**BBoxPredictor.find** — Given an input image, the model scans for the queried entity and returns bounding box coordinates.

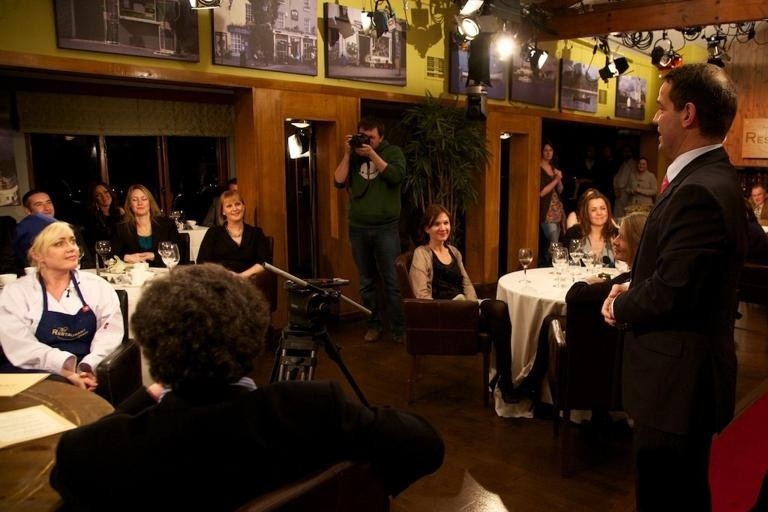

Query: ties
[660,174,670,194]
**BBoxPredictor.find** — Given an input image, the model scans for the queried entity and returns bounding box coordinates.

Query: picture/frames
[53,0,201,67]
[559,56,602,114]
[510,41,557,107]
[447,26,507,99]
[614,71,648,122]
[325,3,406,86]
[212,0,319,78]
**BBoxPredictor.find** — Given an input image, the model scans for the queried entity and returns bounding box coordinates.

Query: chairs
[548,314,627,479]
[97,289,142,404]
[699,377,768,512]
[245,463,364,512]
[393,250,495,409]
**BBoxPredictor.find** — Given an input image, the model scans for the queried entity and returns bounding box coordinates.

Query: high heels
[504,382,541,412]
[490,373,519,404]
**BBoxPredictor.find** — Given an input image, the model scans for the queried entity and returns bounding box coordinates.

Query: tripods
[270,332,377,420]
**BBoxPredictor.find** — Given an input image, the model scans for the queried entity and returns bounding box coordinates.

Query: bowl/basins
[186,221,196,226]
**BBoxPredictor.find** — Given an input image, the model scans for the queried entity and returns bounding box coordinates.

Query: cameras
[350,135,370,148]
[284,278,351,329]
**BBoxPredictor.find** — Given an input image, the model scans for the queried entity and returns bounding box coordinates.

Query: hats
[13,213,58,255]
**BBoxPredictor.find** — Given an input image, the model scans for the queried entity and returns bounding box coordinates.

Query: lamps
[599,42,630,84]
[287,119,311,159]
[529,49,549,72]
[451,18,480,51]
[652,27,685,79]
[459,0,484,17]
[361,0,393,40]
[701,25,731,66]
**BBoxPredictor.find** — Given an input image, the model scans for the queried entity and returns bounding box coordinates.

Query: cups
[157,242,170,269]
[134,262,148,270]
[552,246,568,288]
[161,243,180,271]
[24,267,36,275]
[0,274,16,289]
[126,270,146,288]
[569,239,584,275]
[581,245,594,276]
[549,241,561,256]
[95,240,111,269]
[519,248,533,284]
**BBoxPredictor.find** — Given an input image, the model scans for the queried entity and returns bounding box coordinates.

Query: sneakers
[364,329,388,342]
[394,335,403,347]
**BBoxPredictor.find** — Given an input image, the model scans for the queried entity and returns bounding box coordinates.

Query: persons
[537,141,564,267]
[409,203,523,404]
[565,194,620,268]
[197,190,272,291]
[21,190,54,217]
[110,184,187,267]
[82,181,126,268]
[48,262,446,512]
[750,184,768,229]
[601,63,749,512]
[613,147,637,218]
[510,212,650,413]
[625,156,659,207]
[202,178,237,228]
[565,188,603,230]
[0,212,125,392]
[332,114,407,344]
